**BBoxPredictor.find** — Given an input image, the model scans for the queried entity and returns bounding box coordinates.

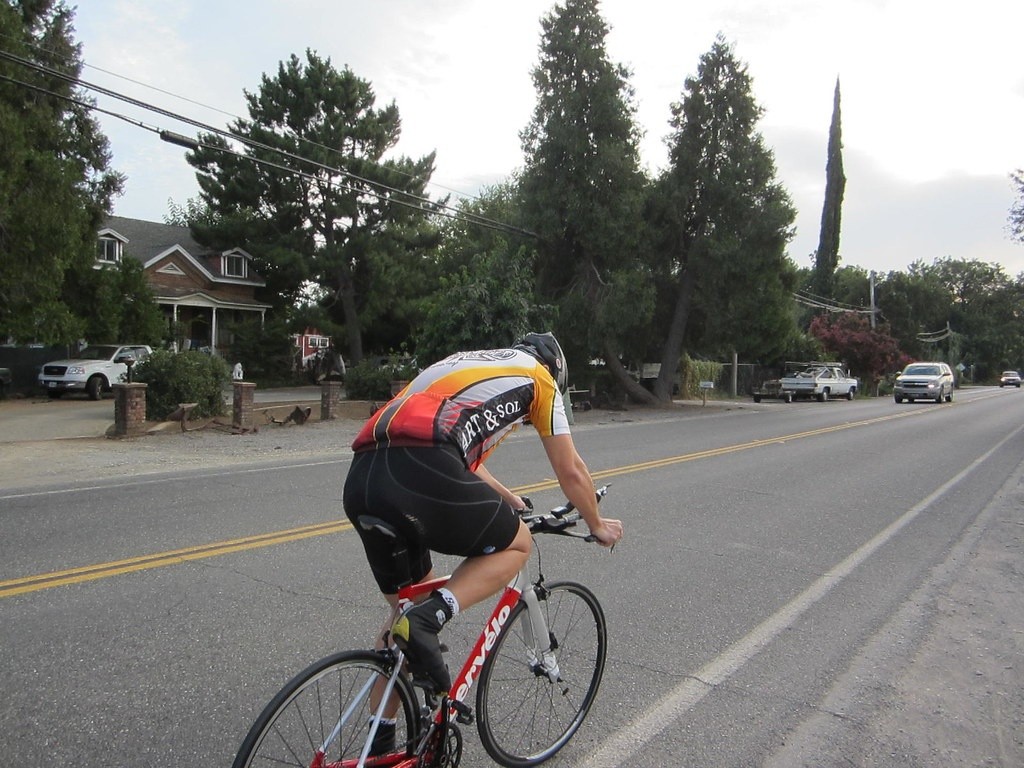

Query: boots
[389,591,454,694]
[360,724,398,768]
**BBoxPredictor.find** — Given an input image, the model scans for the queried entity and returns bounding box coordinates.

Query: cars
[997,369,1021,386]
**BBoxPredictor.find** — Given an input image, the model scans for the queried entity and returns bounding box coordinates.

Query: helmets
[511,331,569,396]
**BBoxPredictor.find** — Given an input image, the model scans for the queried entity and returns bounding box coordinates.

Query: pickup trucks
[37,343,155,402]
[752,367,857,403]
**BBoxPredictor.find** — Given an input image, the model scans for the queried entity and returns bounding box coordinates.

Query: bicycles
[227,482,624,768]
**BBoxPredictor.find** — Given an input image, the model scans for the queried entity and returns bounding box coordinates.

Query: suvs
[892,361,955,403]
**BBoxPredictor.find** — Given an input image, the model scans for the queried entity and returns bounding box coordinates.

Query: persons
[342,331,624,768]
